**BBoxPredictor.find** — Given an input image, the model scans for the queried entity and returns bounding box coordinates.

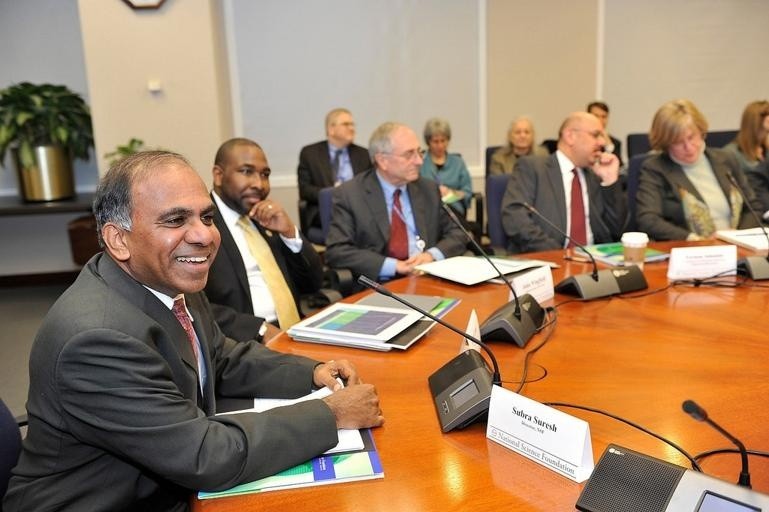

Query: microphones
[575,400,769,512]
[440,202,546,348]
[725,168,768,280]
[518,199,648,301]
[356,275,501,434]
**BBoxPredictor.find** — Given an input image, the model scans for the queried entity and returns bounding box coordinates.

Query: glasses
[381,150,426,160]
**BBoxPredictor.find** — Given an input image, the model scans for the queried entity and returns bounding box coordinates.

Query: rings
[267,205,272,208]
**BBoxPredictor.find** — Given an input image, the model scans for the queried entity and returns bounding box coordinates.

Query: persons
[588,104,623,167]
[324,123,467,297]
[1,151,384,511]
[420,119,472,215]
[724,100,769,174]
[488,119,549,179]
[202,139,324,351]
[634,99,763,241]
[501,112,627,253]
[295,108,372,246]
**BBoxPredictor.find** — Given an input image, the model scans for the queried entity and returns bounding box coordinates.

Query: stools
[68,218,105,266]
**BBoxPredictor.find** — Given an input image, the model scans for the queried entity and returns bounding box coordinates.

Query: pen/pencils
[563,256,593,262]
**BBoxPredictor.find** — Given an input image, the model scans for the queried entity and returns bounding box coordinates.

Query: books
[286,292,462,351]
[197,385,384,501]
[716,226,768,252]
[570,240,669,270]
[415,251,562,287]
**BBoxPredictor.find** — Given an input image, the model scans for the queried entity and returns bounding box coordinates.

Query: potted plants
[0,81,94,202]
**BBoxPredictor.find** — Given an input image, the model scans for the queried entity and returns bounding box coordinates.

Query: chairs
[628,152,655,211]
[0,395,31,512]
[298,199,308,235]
[324,268,354,296]
[486,146,500,176]
[486,174,511,249]
[464,191,483,245]
[626,133,651,156]
[544,139,558,155]
[706,130,738,151]
[298,287,343,318]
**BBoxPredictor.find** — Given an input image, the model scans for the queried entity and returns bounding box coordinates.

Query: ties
[331,149,343,175]
[170,297,199,362]
[566,167,587,249]
[389,188,408,261]
[235,214,301,331]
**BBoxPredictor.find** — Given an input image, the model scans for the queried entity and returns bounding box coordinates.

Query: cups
[621,231,649,272]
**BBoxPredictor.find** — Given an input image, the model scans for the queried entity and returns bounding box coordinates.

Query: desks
[190,239,769,512]
